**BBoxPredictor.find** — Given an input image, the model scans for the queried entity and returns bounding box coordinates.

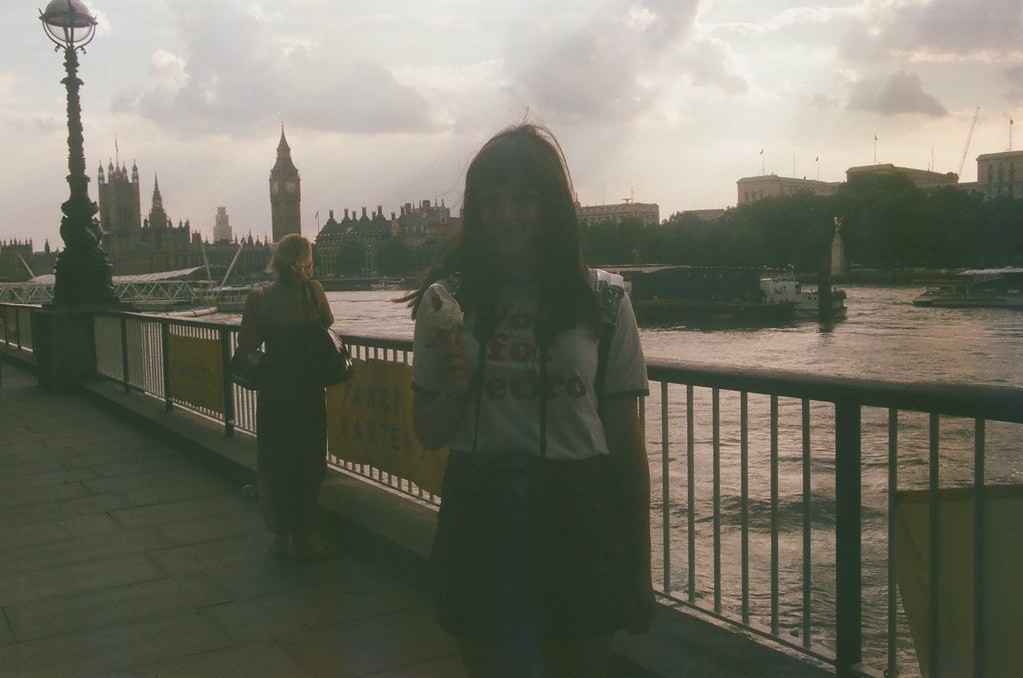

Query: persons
[393,125,650,678]
[238,234,335,561]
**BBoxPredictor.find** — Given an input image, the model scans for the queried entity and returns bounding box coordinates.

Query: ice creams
[423,283,463,344]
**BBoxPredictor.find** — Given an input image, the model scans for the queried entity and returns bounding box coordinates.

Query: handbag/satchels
[227,345,277,392]
[305,279,353,387]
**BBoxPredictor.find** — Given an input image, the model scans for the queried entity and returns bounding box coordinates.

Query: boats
[913,265,1023,309]
[216,279,252,312]
[603,262,794,324]
[759,265,848,315]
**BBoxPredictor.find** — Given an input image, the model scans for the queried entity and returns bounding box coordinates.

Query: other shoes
[293,536,324,554]
[268,541,288,560]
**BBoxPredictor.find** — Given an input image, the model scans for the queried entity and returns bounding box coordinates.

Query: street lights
[37,0,128,311]
